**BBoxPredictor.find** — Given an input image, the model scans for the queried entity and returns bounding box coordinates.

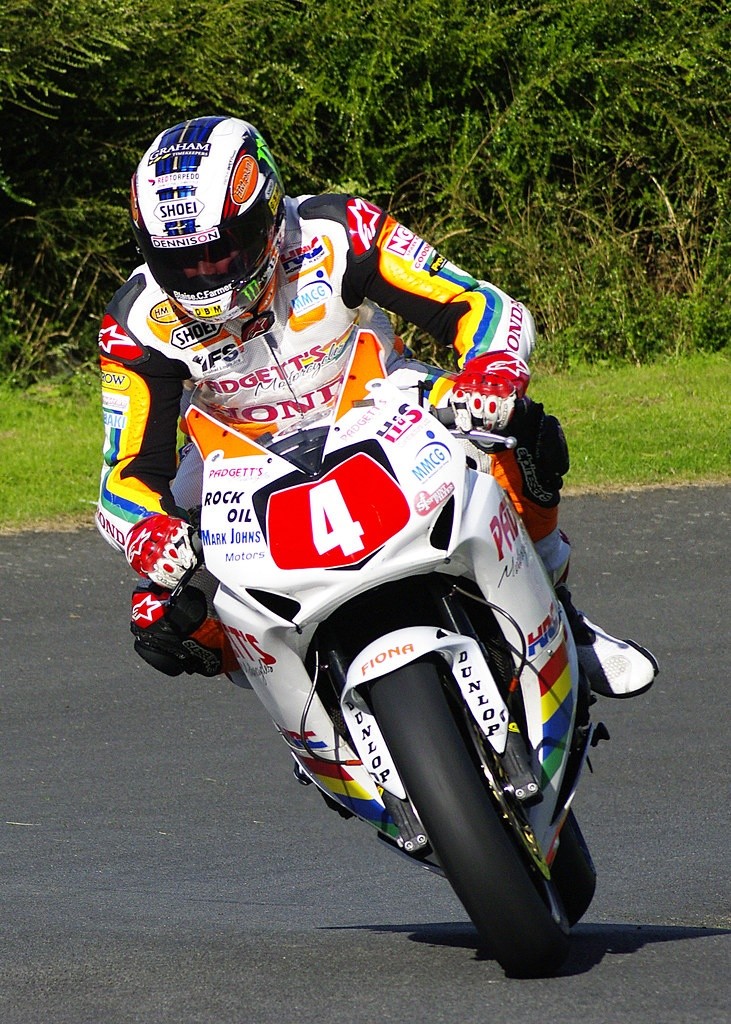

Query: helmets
[130,115,286,325]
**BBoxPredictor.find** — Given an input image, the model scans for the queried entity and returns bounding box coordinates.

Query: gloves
[123,515,203,590]
[447,349,531,432]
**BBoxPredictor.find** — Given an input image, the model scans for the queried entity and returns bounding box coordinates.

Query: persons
[93,111,663,706]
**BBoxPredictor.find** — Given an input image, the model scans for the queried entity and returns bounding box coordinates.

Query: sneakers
[567,612,659,700]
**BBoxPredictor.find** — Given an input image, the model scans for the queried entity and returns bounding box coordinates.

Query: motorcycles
[131,318,613,979]
